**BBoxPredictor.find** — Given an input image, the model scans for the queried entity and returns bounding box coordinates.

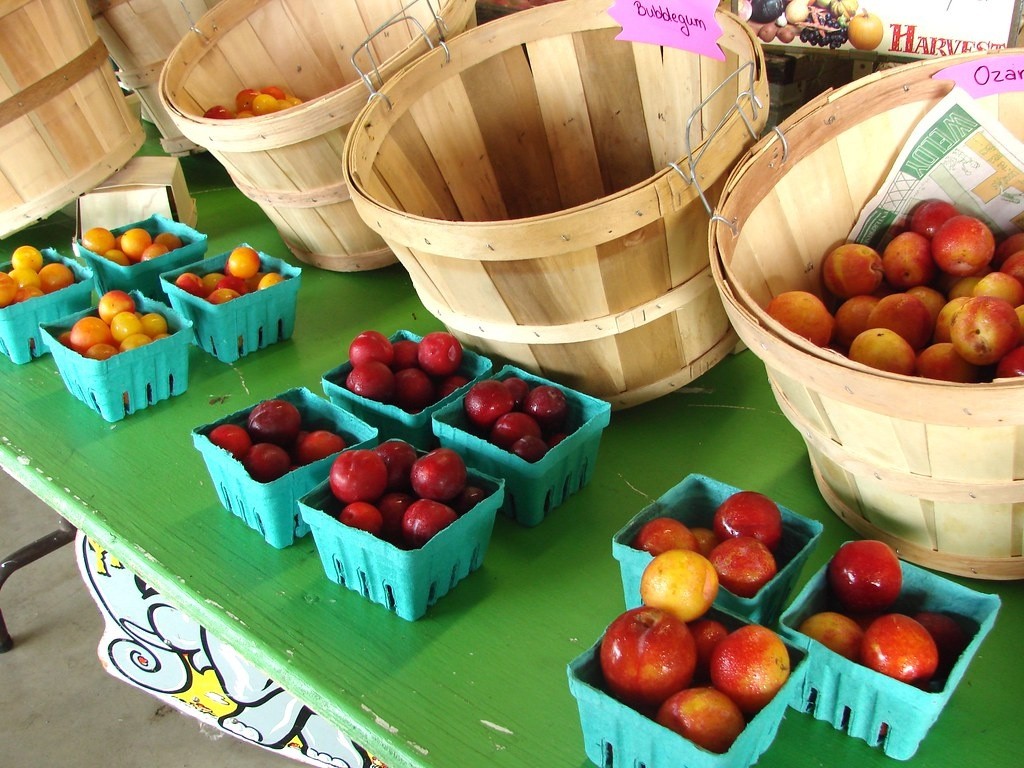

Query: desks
[0,102,1024,768]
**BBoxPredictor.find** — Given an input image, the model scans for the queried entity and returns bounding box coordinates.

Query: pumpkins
[848,7,884,51]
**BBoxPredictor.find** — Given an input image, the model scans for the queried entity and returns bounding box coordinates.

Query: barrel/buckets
[84,0,225,159]
[349,0,770,412]
[0,0,149,242]
[690,49,1024,580]
[157,0,478,273]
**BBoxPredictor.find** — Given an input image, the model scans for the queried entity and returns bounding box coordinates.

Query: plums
[768,202,1024,384]
[204,89,304,117]
[0,228,285,359]
[600,491,964,756]
[211,331,569,546]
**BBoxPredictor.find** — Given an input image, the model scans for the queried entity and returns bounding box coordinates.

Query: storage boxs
[75,158,198,243]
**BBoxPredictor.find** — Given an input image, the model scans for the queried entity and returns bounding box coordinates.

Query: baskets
[611,472,824,627]
[296,438,505,622]
[158,242,302,365]
[566,603,810,768]
[37,288,188,423]
[774,540,1002,761]
[192,385,378,549]
[0,245,93,364]
[321,330,492,454]
[431,364,612,526]
[73,213,206,308]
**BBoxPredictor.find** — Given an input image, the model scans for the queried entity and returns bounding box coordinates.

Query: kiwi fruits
[747,24,795,44]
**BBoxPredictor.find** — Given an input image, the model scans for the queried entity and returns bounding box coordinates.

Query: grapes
[799,13,848,50]
